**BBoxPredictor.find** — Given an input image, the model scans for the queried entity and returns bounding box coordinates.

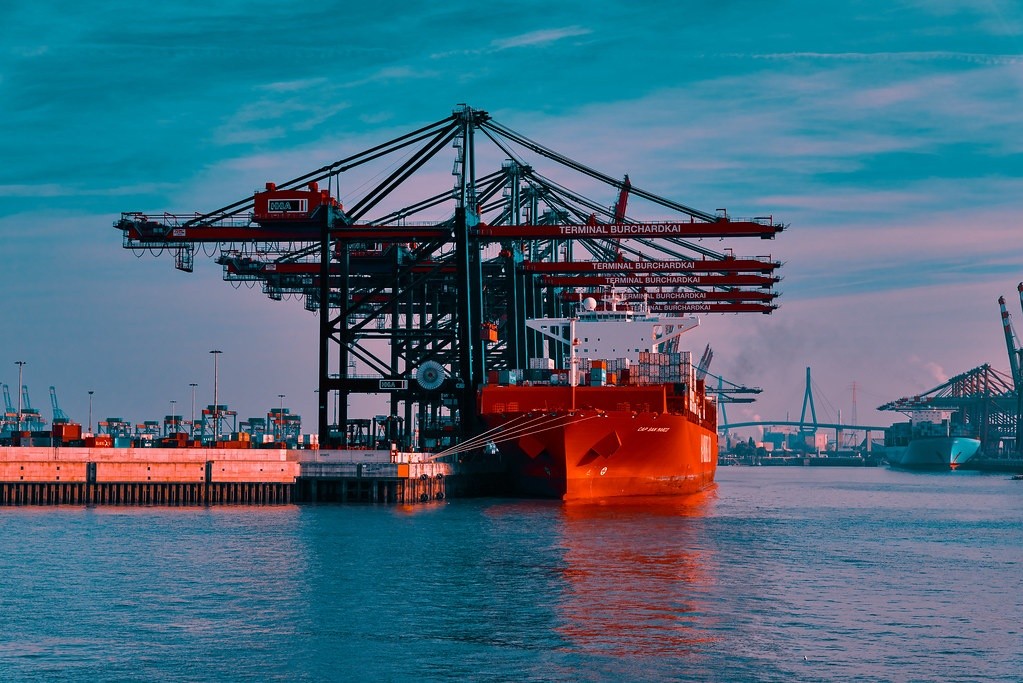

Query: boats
[880,435,981,473]
[476,315,723,506]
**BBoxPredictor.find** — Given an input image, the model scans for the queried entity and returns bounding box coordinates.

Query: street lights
[88,390,94,433]
[170,400,176,433]
[330,373,339,432]
[14,361,26,418]
[278,395,285,436]
[189,383,199,437]
[208,349,223,415]
